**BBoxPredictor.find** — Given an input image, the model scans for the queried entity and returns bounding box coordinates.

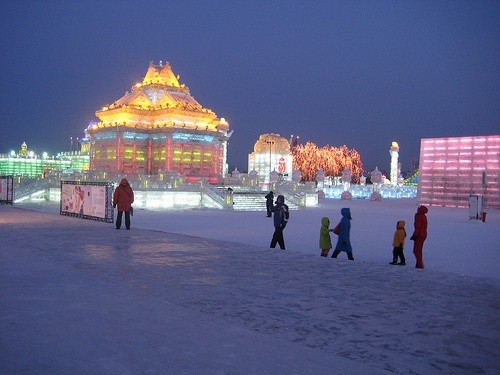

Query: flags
[294,140,297,147]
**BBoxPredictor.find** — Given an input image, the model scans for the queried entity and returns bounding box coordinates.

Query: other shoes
[397,260,406,265]
[116,227,120,229]
[126,228,130,230]
[389,259,398,265]
[266,216,271,217]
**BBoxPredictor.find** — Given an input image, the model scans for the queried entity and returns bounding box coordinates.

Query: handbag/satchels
[130,207,133,217]
[333,223,340,235]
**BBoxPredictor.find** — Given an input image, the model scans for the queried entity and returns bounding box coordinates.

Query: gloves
[344,241,348,247]
[398,243,403,248]
[410,234,417,241]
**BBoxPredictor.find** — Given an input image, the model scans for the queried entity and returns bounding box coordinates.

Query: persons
[319,217,334,257]
[332,207,353,261]
[389,220,407,266]
[113,177,135,230]
[410,205,428,269]
[228,187,235,206]
[265,191,274,218]
[270,195,289,250]
